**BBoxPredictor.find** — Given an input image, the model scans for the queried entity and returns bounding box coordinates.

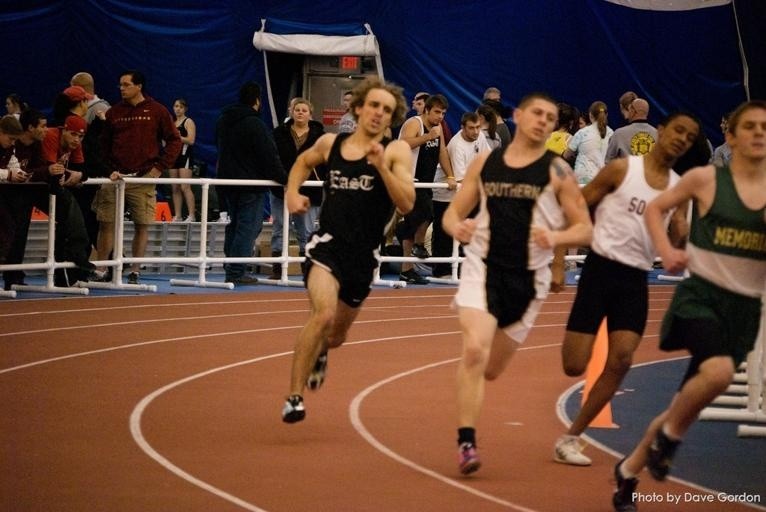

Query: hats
[62,85,95,102]
[57,115,88,134]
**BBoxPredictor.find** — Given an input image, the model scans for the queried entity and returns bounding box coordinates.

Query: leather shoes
[224,275,259,286]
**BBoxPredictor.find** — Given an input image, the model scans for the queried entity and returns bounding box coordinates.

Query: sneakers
[612,456,642,512]
[399,268,430,285]
[128,271,140,284]
[551,434,593,466]
[281,393,307,424]
[643,420,684,484]
[455,443,482,475]
[305,350,328,391]
[409,243,431,259]
[184,214,195,224]
[172,216,182,222]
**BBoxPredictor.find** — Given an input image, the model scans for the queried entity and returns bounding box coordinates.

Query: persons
[216,82,289,285]
[413,94,454,257]
[480,99,511,144]
[483,87,501,102]
[561,100,614,185]
[612,102,766,511]
[416,94,453,146]
[35,116,97,287]
[165,98,197,222]
[619,92,639,124]
[342,91,354,112]
[604,99,659,164]
[706,113,733,165]
[574,113,590,129]
[550,110,706,465]
[719,113,736,170]
[398,95,457,284]
[1,110,71,291]
[339,98,367,133]
[544,102,580,158]
[406,92,430,119]
[70,73,130,282]
[474,88,502,117]
[282,97,320,240]
[475,105,501,150]
[0,116,34,294]
[579,110,591,129]
[91,70,183,285]
[432,112,492,275]
[268,99,327,284]
[443,94,593,473]
[5,93,32,131]
[281,76,416,423]
[62,86,110,280]
